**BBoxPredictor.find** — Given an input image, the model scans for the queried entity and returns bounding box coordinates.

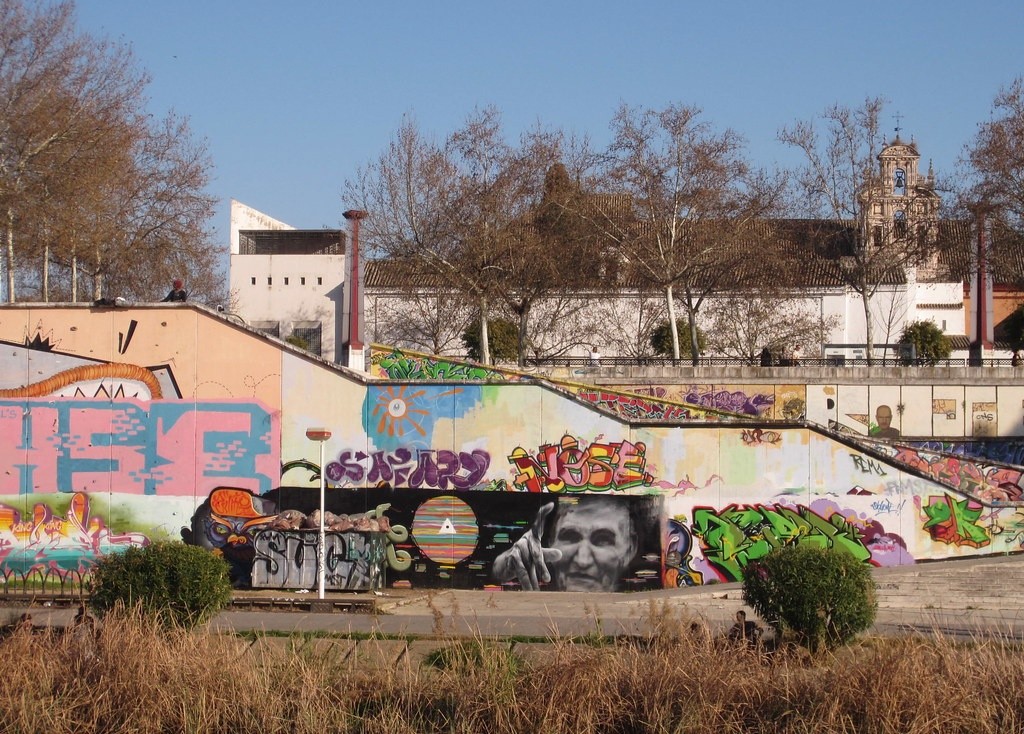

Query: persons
[159,279,188,303]
[724,610,765,650]
